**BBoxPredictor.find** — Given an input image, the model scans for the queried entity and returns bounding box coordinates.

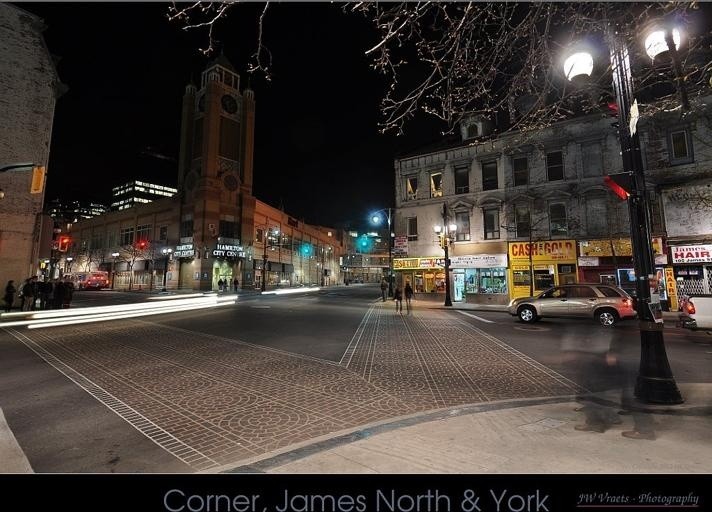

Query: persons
[380,280,389,303]
[217,279,224,290]
[606,299,661,441]
[573,342,633,431]
[234,278,239,292]
[393,283,404,316]
[230,279,233,291]
[4,273,76,315]
[223,279,228,291]
[403,283,414,316]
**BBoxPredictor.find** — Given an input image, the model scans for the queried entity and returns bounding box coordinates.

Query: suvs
[79,273,108,291]
[506,283,637,327]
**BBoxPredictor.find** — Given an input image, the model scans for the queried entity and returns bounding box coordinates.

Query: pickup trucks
[676,293,712,336]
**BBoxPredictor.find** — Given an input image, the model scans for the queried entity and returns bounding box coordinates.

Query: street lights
[162,248,172,292]
[109,252,120,291]
[563,23,684,402]
[361,207,393,297]
[433,200,459,306]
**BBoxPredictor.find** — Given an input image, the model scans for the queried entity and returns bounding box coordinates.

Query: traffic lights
[59,237,67,252]
[438,235,441,246]
[603,170,638,203]
[304,243,309,254]
[140,240,145,249]
